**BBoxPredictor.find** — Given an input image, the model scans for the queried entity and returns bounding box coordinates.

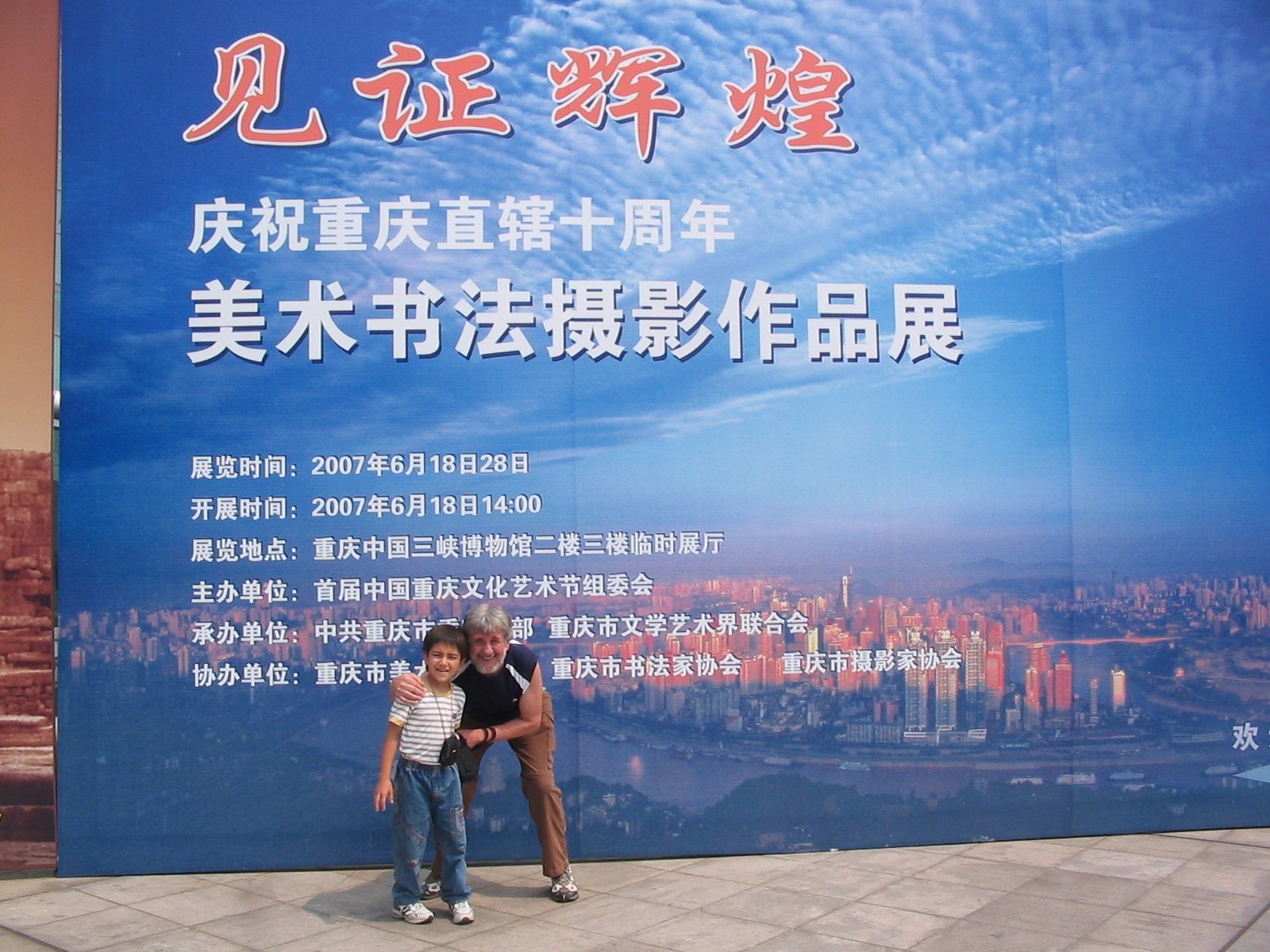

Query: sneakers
[551,864,578,902]
[392,898,434,925]
[449,900,474,925]
[417,873,441,900]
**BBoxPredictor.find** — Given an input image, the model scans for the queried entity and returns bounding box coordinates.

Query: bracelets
[488,727,496,743]
[483,728,488,744]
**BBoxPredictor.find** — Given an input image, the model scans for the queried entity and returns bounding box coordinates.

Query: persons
[375,625,473,926]
[391,605,578,902]
